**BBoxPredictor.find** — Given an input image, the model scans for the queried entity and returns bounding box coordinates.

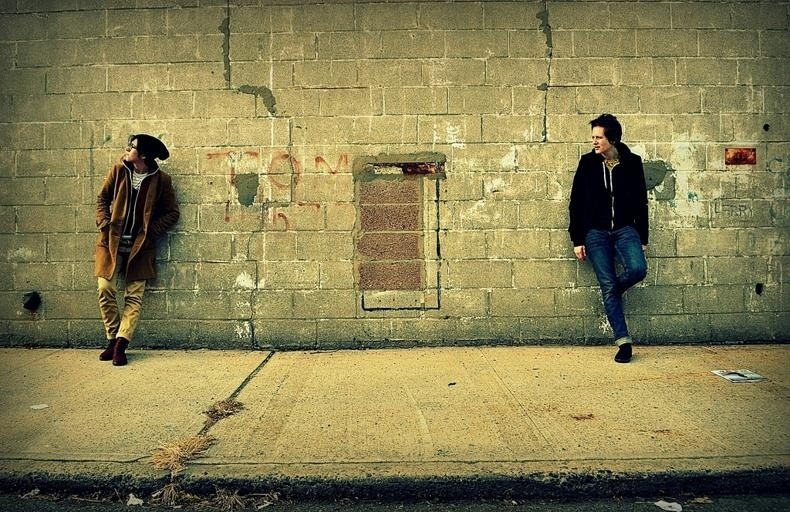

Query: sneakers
[615,343,632,363]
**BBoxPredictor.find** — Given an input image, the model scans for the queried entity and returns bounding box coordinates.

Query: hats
[131,134,169,160]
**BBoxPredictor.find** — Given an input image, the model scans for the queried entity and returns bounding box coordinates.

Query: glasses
[129,143,137,149]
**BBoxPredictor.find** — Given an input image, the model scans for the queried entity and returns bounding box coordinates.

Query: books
[712,369,764,382]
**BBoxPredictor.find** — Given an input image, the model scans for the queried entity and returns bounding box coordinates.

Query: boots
[99,338,117,361]
[112,337,129,366]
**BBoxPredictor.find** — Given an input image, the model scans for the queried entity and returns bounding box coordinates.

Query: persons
[94,134,179,366]
[567,113,649,362]
